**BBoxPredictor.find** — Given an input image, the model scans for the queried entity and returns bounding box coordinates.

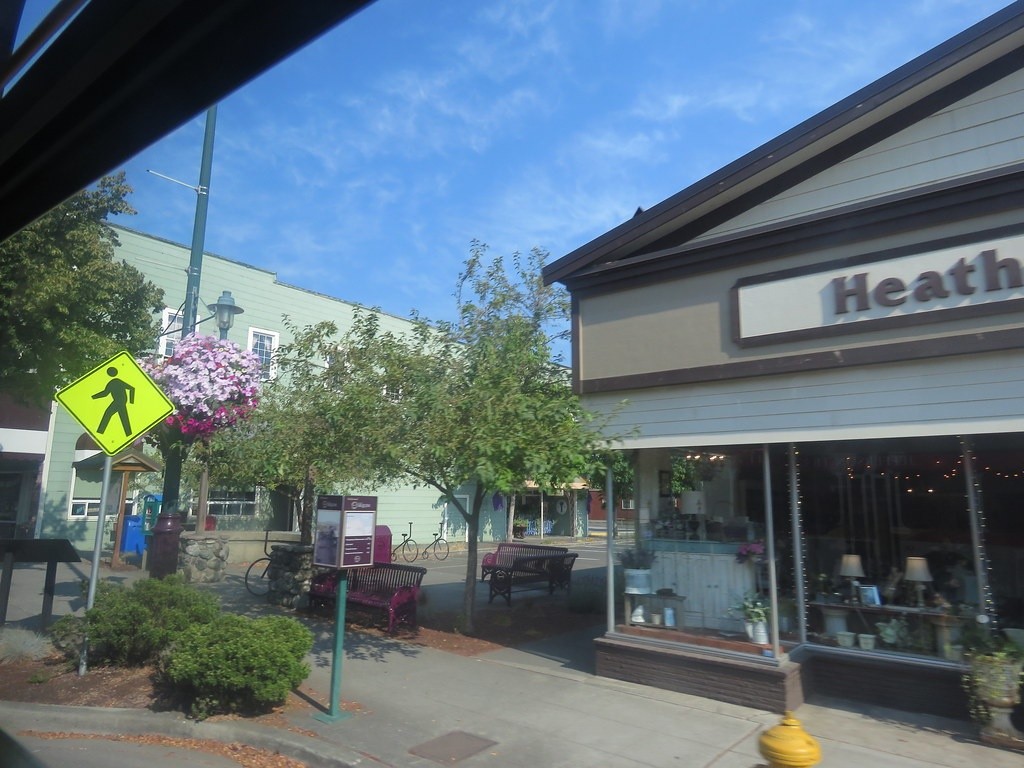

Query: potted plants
[958,639,1024,749]
[615,540,659,595]
[721,590,795,644]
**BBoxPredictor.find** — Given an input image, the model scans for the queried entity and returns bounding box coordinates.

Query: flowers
[735,543,767,564]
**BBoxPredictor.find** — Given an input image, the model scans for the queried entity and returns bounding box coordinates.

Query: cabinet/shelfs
[621,593,687,632]
[644,552,757,631]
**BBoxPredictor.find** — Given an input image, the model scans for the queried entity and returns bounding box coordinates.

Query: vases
[651,611,663,625]
[835,630,857,647]
[856,633,877,650]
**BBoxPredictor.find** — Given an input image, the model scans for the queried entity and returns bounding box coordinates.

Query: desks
[810,603,975,659]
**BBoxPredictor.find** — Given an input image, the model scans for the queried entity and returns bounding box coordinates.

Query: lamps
[902,555,936,607]
[839,553,867,605]
[681,489,708,540]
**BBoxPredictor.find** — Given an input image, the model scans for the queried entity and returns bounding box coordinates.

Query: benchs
[480,538,579,606]
[304,561,428,635]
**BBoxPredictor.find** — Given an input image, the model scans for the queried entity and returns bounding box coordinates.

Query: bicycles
[421,523,450,561]
[391,522,418,562]
[243,556,273,597]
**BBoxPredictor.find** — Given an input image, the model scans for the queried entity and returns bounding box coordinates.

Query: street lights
[144,289,242,580]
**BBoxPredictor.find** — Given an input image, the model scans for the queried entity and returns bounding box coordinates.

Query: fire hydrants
[753,708,822,768]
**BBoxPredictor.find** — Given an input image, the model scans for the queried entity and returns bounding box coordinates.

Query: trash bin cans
[374,525,393,565]
[120,514,145,554]
[206,516,217,531]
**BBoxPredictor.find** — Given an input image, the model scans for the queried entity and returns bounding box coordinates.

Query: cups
[838,631,857,647]
[858,633,877,650]
[650,613,661,625]
[663,607,676,626]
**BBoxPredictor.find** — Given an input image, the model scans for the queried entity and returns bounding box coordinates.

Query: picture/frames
[658,470,673,498]
